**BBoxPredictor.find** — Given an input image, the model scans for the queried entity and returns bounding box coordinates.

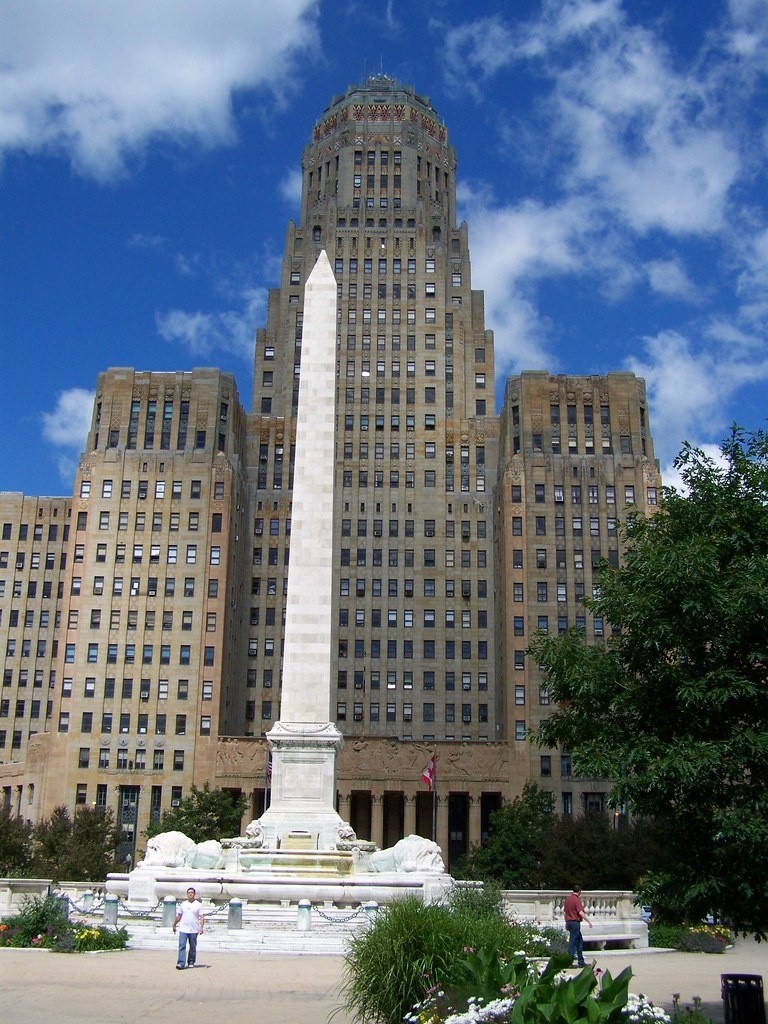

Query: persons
[564,884,592,968]
[173,888,204,970]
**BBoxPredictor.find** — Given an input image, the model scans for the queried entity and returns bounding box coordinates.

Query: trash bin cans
[719,973,768,1024]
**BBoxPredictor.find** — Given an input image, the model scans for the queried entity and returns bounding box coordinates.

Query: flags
[421,754,435,792]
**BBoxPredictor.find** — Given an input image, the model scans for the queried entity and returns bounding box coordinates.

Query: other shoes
[578,964,588,967]
[565,965,577,969]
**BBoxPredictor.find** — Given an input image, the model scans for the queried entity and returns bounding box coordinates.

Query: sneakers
[189,964,194,968]
[176,965,184,969]
